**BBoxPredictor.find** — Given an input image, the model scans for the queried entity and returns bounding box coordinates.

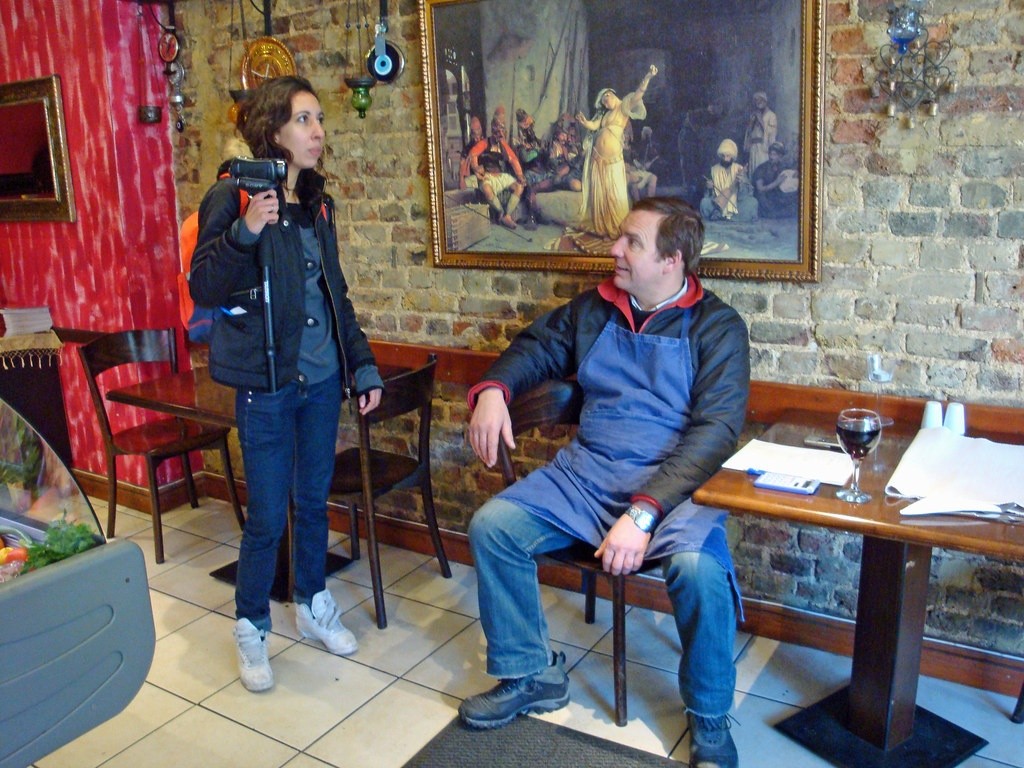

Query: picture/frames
[0,74,76,223]
[418,0,826,283]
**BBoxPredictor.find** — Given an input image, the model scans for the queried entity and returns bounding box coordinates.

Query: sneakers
[459,650,573,728]
[232,617,274,691]
[294,589,358,656]
[682,703,741,768]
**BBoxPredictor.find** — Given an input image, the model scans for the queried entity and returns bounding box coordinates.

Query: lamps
[861,1,958,129]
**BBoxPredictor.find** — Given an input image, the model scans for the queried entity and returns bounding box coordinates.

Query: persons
[188,76,382,691]
[458,198,751,768]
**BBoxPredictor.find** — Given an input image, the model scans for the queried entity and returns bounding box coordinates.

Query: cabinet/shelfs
[0,329,72,466]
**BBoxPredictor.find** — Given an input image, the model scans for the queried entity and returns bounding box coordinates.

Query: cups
[920,401,943,431]
[944,402,966,436]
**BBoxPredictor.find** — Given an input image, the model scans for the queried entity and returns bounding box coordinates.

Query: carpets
[401,714,690,768]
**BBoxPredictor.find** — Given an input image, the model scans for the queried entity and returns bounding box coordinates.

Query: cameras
[231,157,288,200]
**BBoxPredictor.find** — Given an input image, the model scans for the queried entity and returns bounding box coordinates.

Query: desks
[106,363,414,602]
[692,407,1024,768]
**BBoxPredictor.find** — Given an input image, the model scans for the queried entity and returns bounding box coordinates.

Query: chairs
[76,326,246,566]
[497,380,663,728]
[286,352,452,630]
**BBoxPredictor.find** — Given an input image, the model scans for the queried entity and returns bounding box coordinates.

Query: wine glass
[834,408,882,503]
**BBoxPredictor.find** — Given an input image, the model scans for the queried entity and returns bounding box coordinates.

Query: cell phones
[804,435,843,451]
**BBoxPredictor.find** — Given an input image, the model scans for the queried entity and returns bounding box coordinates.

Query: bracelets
[625,507,656,532]
